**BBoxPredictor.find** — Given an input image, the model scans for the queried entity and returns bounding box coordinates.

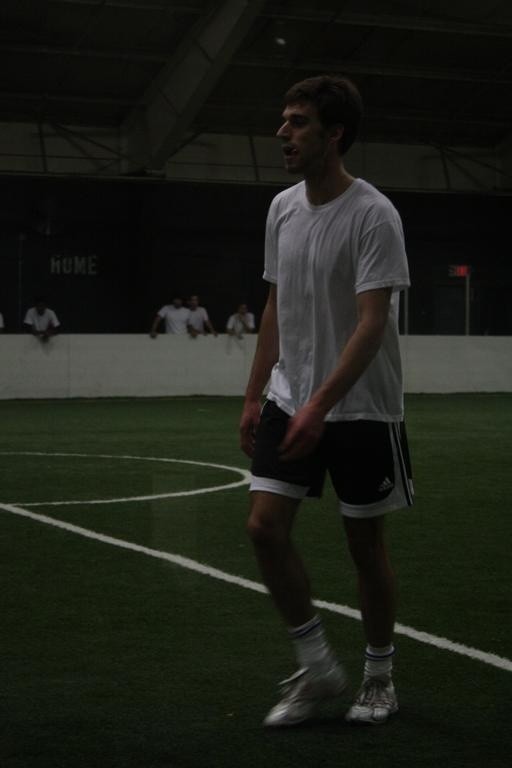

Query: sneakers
[262,659,347,728]
[346,672,401,726]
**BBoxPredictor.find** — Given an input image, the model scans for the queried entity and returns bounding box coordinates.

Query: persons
[148,291,219,339]
[21,298,63,343]
[235,72,413,730]
[226,302,256,338]
[0,311,6,333]
